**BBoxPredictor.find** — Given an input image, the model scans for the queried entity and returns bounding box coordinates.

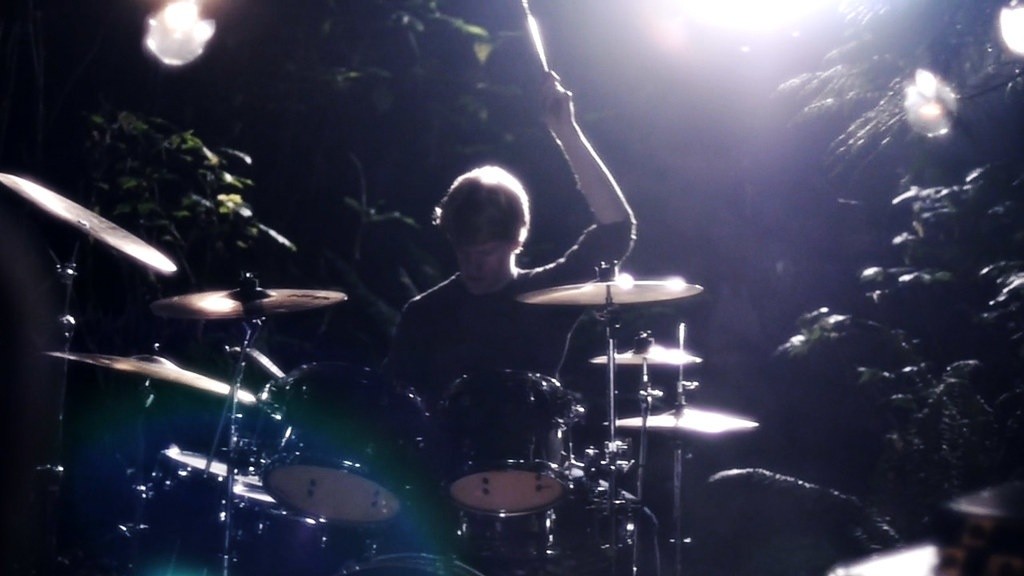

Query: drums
[323,539,488,574]
[248,361,431,524]
[142,449,243,557]
[440,366,579,521]
[220,471,333,575]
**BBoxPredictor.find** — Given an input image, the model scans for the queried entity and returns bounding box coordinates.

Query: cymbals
[0,174,180,277]
[141,285,349,323]
[38,345,257,410]
[515,277,706,306]
[604,408,760,436]
[590,342,703,368]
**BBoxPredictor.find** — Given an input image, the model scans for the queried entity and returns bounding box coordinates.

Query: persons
[379,71,638,576]
